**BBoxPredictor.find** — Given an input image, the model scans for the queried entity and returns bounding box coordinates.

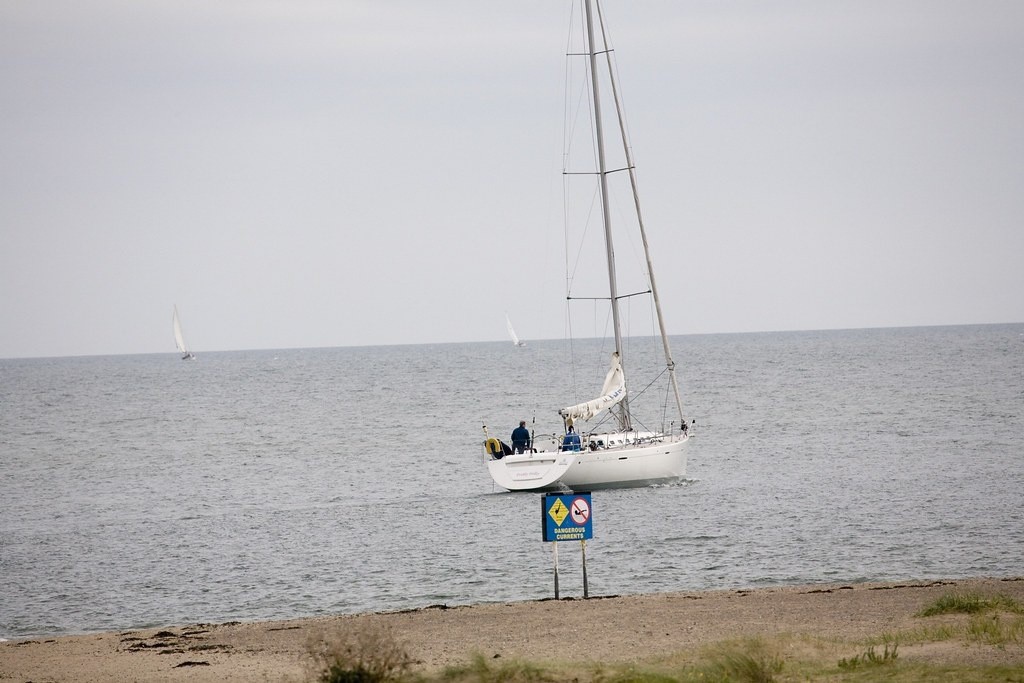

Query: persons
[511,421,530,455]
[563,413,576,434]
[680,419,688,436]
[581,439,605,451]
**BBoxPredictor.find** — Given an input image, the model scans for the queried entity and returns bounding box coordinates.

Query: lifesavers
[486,438,500,454]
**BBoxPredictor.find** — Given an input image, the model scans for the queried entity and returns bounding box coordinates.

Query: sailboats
[478,1,700,495]
[504,314,527,349]
[172,311,196,361]
